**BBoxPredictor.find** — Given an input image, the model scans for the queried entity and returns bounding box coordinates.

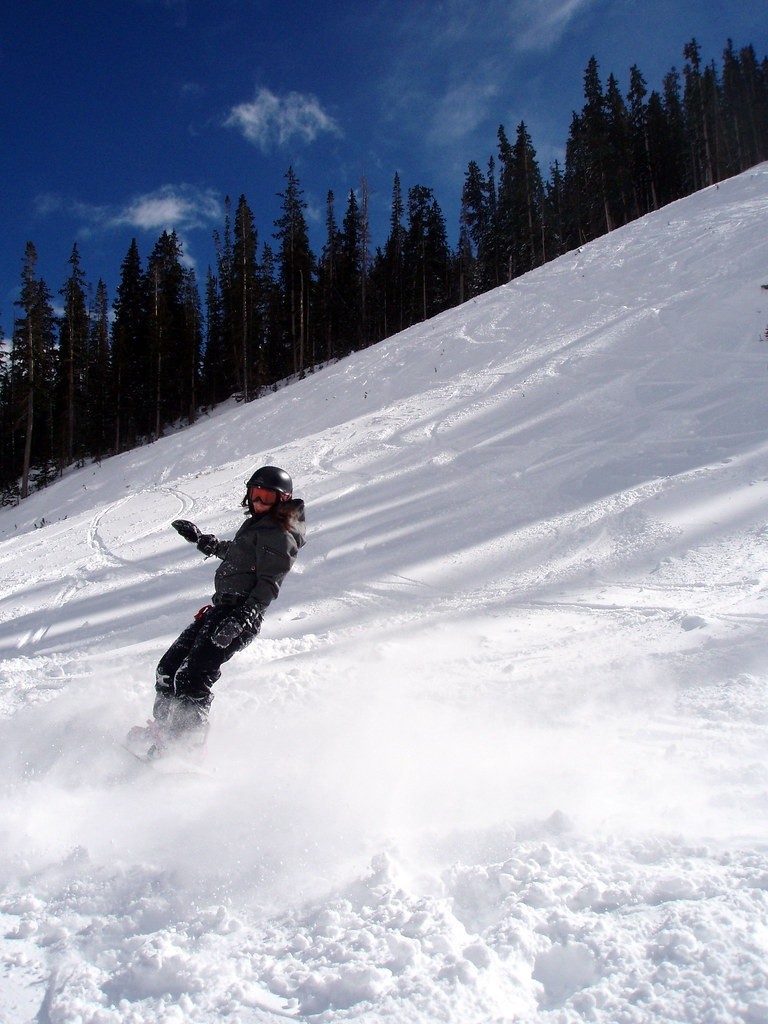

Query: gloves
[212,603,254,649]
[172,519,217,557]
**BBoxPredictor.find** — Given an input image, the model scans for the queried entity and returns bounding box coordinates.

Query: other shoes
[128,719,165,741]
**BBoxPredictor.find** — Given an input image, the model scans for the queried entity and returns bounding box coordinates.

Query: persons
[147,467,305,753]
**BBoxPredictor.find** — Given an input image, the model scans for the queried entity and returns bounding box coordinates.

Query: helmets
[247,465,293,497]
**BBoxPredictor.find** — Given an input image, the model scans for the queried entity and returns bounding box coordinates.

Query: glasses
[247,486,281,505]
